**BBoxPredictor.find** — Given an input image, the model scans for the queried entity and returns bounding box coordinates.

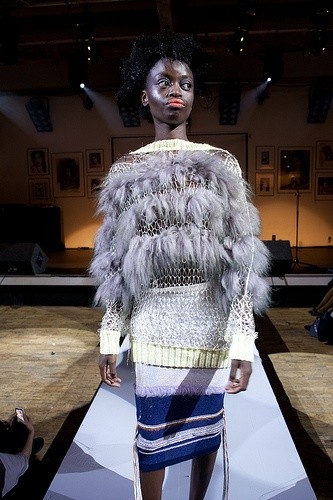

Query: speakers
[261,239,292,277]
[0,243,50,276]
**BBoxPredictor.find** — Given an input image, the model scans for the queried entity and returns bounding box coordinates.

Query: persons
[86,29,270,500]
[304,278,333,345]
[30,151,46,174]
[0,411,35,497]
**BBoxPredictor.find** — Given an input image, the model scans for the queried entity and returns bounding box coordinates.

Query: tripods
[291,181,320,272]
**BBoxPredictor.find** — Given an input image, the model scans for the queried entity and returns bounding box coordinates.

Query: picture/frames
[277,146,313,194]
[87,175,105,198]
[50,151,85,197]
[85,148,105,172]
[315,140,333,170]
[255,145,275,170]
[315,172,333,201]
[255,172,274,196]
[28,177,51,204]
[26,146,49,175]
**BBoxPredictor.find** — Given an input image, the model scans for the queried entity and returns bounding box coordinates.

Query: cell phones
[15,408,24,422]
